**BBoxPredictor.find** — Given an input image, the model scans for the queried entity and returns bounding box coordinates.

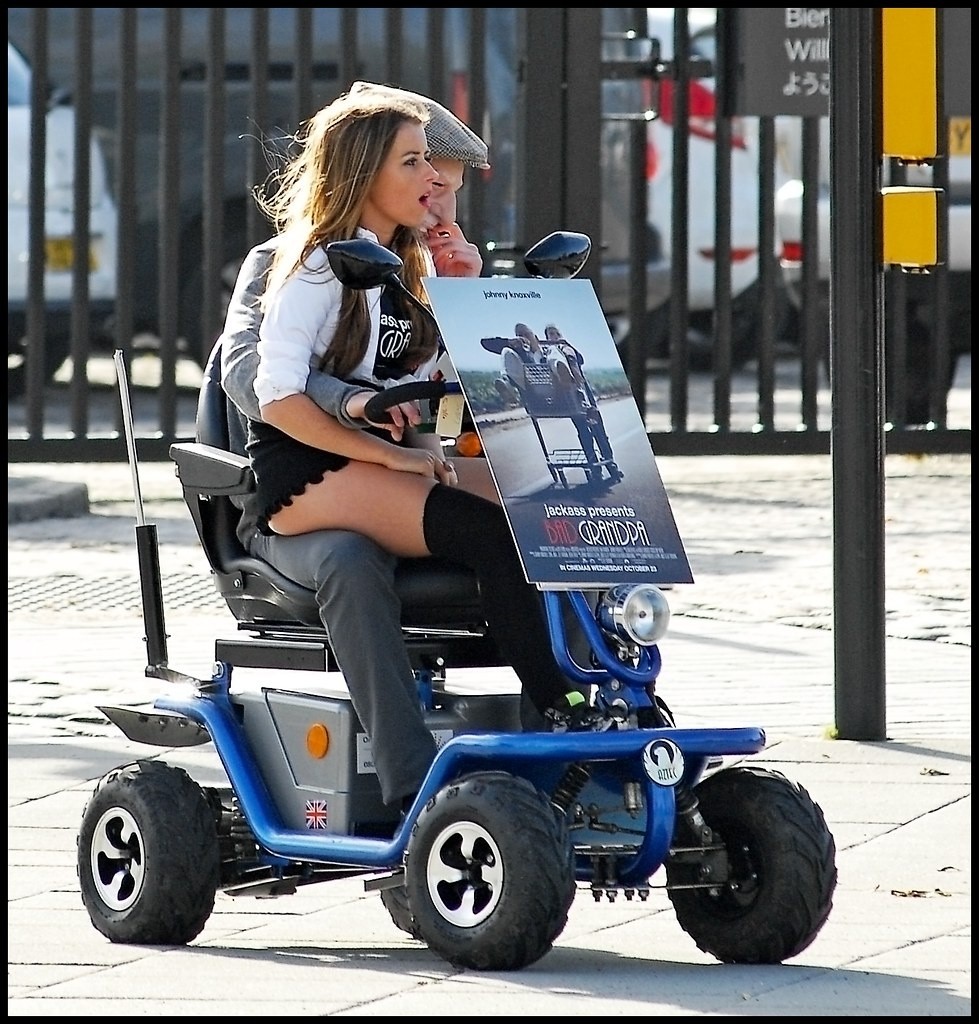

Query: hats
[350,80,489,172]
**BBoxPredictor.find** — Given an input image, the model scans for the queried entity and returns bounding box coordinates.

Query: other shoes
[595,473,602,483]
[613,470,623,480]
[546,691,615,736]
[494,378,521,404]
[575,411,589,425]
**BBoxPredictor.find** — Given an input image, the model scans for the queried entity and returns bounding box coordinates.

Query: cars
[6,44,122,405]
[4,6,973,421]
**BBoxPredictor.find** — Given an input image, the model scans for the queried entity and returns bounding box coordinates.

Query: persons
[243,82,595,732]
[474,322,622,488]
[223,78,608,828]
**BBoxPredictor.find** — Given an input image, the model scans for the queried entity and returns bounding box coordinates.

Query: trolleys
[509,338,621,498]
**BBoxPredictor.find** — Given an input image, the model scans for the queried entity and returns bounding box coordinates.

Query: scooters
[73,233,839,971]
